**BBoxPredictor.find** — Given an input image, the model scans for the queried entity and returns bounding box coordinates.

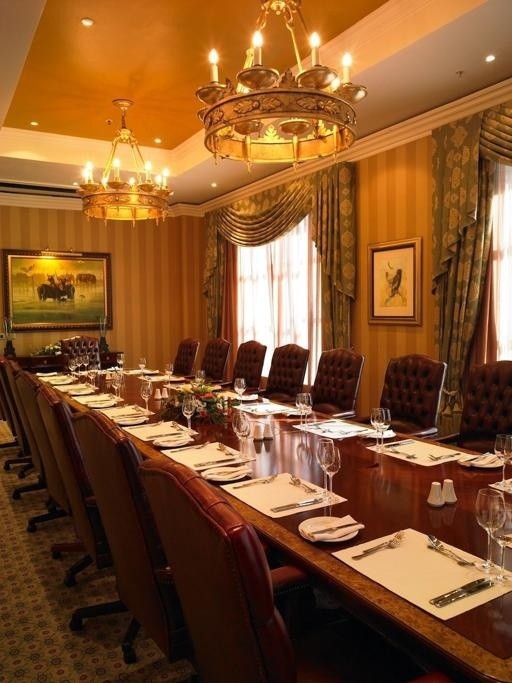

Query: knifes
[310,521,363,532]
[428,577,485,603]
[434,581,494,608]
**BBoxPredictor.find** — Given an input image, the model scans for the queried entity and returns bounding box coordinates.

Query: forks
[351,532,401,561]
[37,372,73,385]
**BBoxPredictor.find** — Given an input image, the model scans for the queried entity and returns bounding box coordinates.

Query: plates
[115,416,146,425]
[89,401,118,409]
[202,466,246,481]
[298,516,358,542]
[153,437,188,448]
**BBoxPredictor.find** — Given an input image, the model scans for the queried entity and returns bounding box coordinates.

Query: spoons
[426,534,474,566]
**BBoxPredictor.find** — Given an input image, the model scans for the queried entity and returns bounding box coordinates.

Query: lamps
[196,0,367,174]
[76,99,169,228]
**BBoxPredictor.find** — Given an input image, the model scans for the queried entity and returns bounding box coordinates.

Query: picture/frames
[368,235,422,326]
[0,248,113,333]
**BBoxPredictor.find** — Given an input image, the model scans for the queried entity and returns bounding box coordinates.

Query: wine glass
[234,378,247,404]
[183,394,197,428]
[319,447,341,492]
[491,504,511,585]
[296,393,305,425]
[315,438,335,489]
[375,409,391,444]
[234,417,250,454]
[504,435,512,492]
[140,381,153,410]
[231,412,246,452]
[182,400,195,431]
[494,434,508,487]
[476,489,507,572]
[370,408,385,445]
[301,392,313,423]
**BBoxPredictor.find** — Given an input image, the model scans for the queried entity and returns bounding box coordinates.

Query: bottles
[427,482,445,507]
[441,479,458,505]
